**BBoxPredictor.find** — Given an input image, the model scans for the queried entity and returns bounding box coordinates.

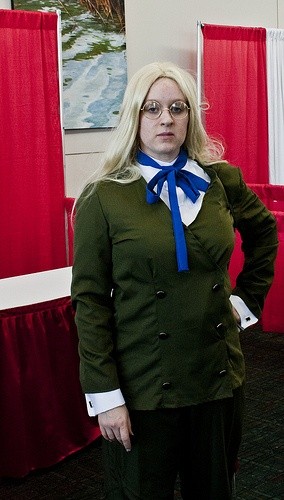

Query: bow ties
[142,165,208,272]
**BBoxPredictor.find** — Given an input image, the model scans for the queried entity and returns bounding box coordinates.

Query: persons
[71,63,278,500]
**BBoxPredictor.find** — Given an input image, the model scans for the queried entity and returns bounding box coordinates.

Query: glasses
[140,100,191,119]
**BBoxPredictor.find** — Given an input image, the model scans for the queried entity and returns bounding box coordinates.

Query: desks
[0,265,102,478]
